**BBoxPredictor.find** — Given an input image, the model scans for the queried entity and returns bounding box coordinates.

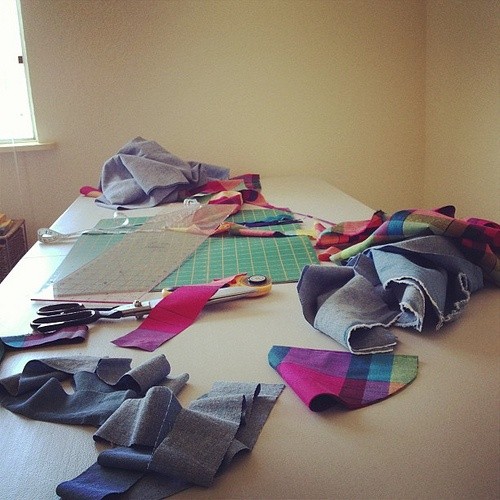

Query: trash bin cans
[0,212,28,282]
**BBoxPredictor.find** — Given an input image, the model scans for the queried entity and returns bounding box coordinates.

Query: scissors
[31,285,258,331]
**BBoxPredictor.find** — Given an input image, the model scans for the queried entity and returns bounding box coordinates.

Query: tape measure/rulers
[36,197,204,243]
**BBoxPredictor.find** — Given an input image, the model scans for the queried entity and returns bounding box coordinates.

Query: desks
[1,169,500,500]
